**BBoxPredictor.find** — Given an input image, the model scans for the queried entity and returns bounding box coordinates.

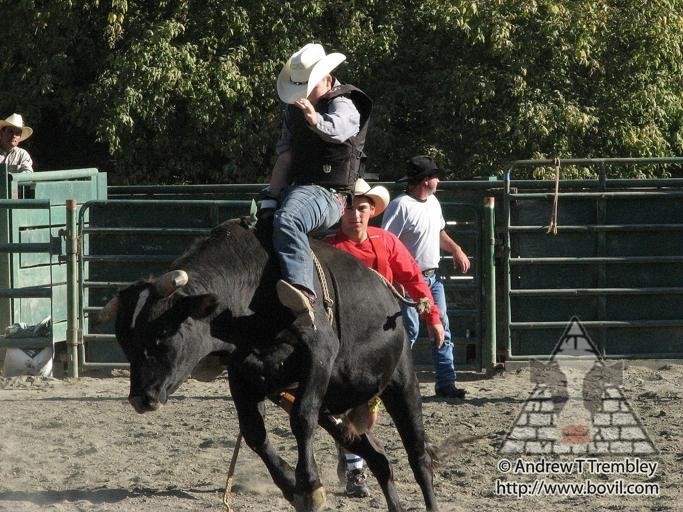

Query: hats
[0,113,33,142]
[355,178,390,218]
[393,155,452,181]
[276,43,347,104]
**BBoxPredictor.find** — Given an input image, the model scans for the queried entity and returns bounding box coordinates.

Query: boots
[436,383,464,398]
[275,279,316,327]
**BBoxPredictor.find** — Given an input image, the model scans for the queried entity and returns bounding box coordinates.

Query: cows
[99,217,438,512]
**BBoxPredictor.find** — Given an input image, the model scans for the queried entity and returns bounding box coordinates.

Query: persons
[380,155,471,397]
[320,178,445,498]
[256,43,373,328]
[0,113,34,173]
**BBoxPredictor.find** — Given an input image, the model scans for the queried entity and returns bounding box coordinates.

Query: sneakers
[337,453,369,497]
